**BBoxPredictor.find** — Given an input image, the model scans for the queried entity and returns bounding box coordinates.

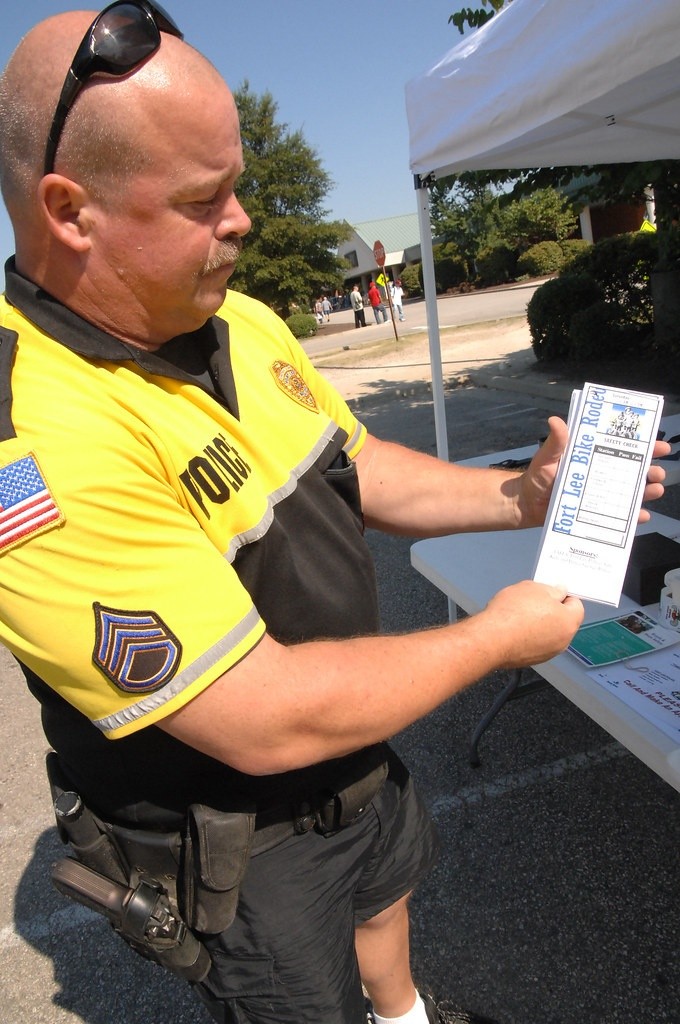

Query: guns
[48,854,213,984]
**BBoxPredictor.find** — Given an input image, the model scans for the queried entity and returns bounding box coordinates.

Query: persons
[314,279,407,328]
[0,1,673,1024]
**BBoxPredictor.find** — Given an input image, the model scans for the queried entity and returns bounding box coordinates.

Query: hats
[395,280,401,286]
[370,282,375,287]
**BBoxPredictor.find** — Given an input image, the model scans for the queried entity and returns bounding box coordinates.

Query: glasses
[43,1,184,175]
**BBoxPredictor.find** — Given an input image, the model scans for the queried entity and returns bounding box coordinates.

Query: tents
[405,0,679,624]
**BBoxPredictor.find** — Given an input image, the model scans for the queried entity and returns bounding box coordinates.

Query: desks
[410,413,680,793]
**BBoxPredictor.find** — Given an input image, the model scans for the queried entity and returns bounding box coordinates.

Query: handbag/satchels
[317,313,322,319]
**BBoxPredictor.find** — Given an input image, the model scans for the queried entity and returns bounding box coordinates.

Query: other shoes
[401,318,406,322]
[367,991,476,1024]
[385,319,391,324]
